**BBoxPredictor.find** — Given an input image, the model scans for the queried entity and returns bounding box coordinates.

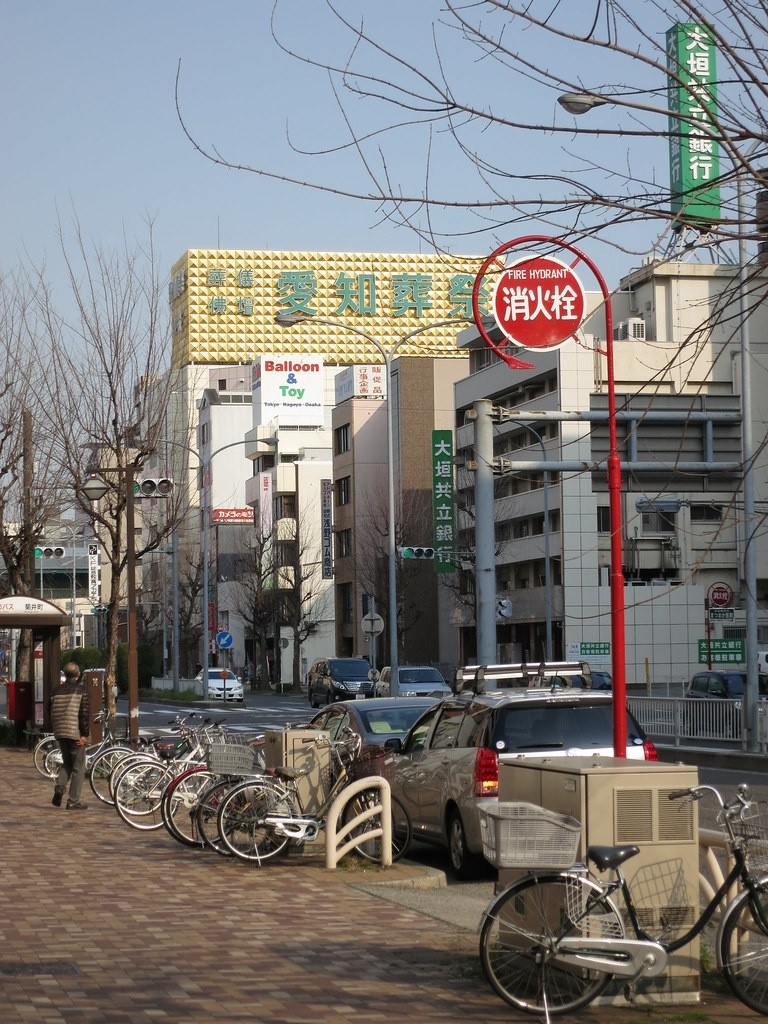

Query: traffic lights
[399,547,435,559]
[131,478,175,497]
[35,546,66,559]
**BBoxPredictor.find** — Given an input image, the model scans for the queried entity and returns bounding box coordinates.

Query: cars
[194,667,245,704]
[377,659,660,872]
[679,671,768,741]
[376,665,453,701]
[301,695,446,791]
[305,656,378,707]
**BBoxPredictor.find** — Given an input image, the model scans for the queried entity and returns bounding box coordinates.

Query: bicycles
[33,708,131,782]
[473,782,767,1024]
[89,711,412,865]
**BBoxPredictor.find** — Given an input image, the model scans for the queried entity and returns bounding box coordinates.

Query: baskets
[476,802,584,869]
[716,801,768,870]
[209,743,255,775]
[331,745,396,786]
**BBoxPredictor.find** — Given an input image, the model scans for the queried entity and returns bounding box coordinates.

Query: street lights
[124,435,280,702]
[20,479,110,598]
[557,92,758,757]
[275,314,496,699]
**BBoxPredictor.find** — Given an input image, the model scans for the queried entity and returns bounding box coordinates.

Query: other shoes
[52,790,63,807]
[67,803,89,809]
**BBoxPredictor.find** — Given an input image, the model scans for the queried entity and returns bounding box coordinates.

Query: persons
[49,661,90,809]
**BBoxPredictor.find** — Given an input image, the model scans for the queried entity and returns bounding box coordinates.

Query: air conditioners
[613,318,646,342]
